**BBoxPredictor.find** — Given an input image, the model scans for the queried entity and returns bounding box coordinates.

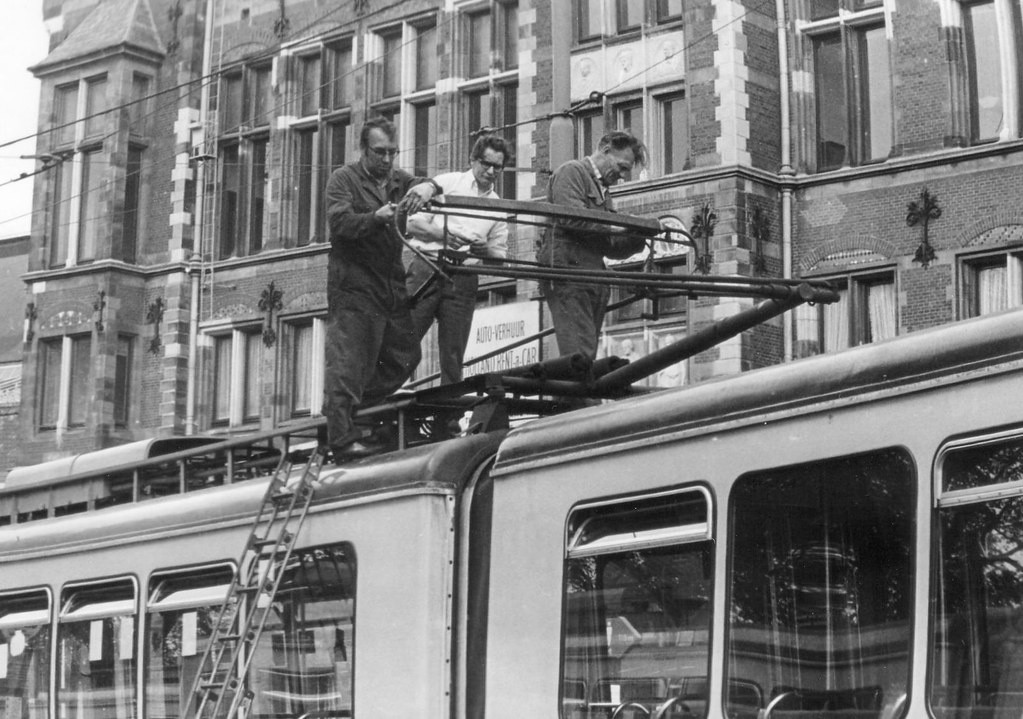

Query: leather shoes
[331,442,383,465]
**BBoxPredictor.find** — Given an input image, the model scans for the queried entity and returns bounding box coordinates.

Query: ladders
[182,442,328,719]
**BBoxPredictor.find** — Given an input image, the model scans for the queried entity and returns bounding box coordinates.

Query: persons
[400,136,509,437]
[537,133,654,410]
[324,115,444,466]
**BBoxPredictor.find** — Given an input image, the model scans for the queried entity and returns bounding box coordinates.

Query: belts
[418,249,459,263]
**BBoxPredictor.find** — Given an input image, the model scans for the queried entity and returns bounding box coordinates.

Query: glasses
[476,158,503,172]
[367,145,399,157]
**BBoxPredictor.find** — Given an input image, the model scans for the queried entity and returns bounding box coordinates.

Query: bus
[0,194,1023,719]
